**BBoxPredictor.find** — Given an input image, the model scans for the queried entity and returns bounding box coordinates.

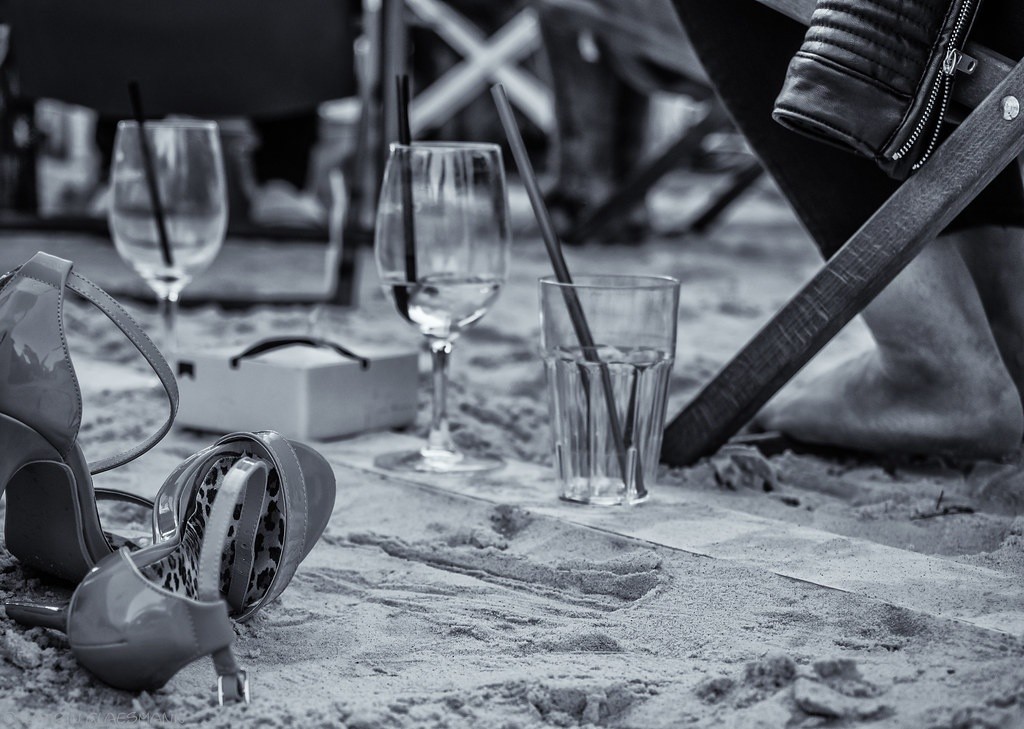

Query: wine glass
[110,122,228,373]
[375,141,512,477]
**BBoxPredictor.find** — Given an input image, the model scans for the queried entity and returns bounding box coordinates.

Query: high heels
[5,430,338,708]
[0,252,181,588]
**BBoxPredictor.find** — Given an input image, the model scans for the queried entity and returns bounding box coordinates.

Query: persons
[674,1,1024,468]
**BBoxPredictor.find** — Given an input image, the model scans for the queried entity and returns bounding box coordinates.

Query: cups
[540,274,681,505]
